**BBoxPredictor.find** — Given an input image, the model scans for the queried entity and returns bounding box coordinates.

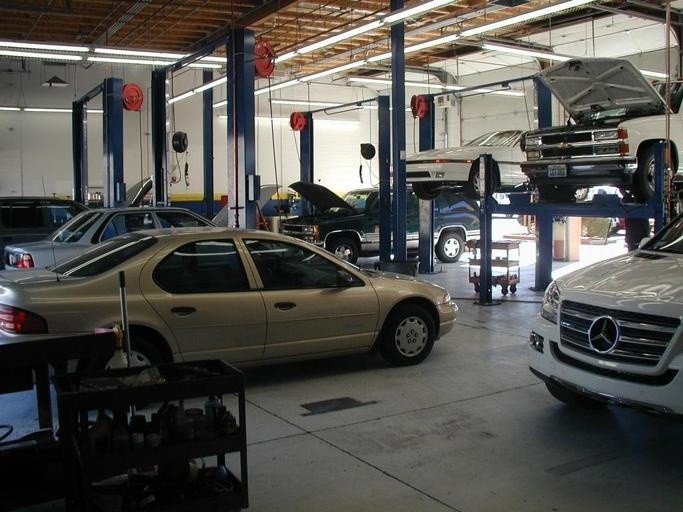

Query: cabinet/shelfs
[51,357,248,512]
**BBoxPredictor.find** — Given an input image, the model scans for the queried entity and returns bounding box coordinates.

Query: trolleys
[465,237,522,296]
[48,354,250,511]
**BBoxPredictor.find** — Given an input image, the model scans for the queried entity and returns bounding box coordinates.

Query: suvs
[0,175,153,269]
[278,179,483,268]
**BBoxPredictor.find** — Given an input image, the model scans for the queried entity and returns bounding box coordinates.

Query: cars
[0,224,460,415]
[404,129,534,202]
[0,184,282,271]
[524,213,683,425]
[520,57,683,204]
